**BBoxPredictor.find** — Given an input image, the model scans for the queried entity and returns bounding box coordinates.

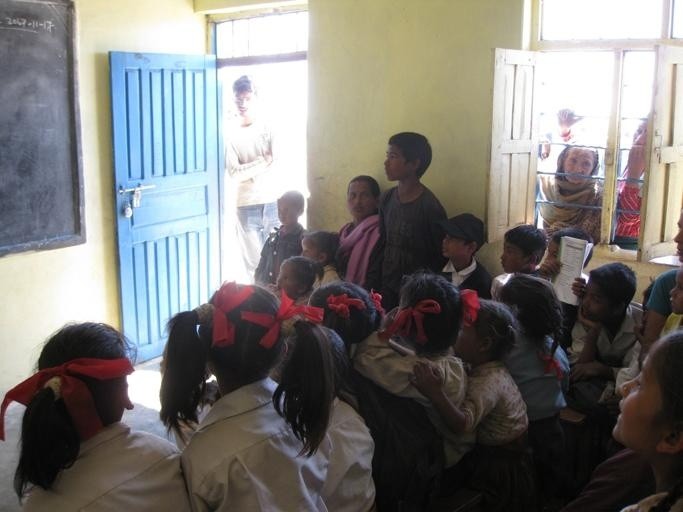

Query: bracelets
[624,185,640,189]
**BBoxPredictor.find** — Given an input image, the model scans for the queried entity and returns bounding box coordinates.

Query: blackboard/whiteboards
[0,0,87,259]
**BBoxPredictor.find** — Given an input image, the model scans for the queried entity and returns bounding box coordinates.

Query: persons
[365,132,448,313]
[160,208,683,512]
[331,175,384,291]
[254,191,307,288]
[532,108,648,237]
[12,322,191,511]
[223,74,283,254]
